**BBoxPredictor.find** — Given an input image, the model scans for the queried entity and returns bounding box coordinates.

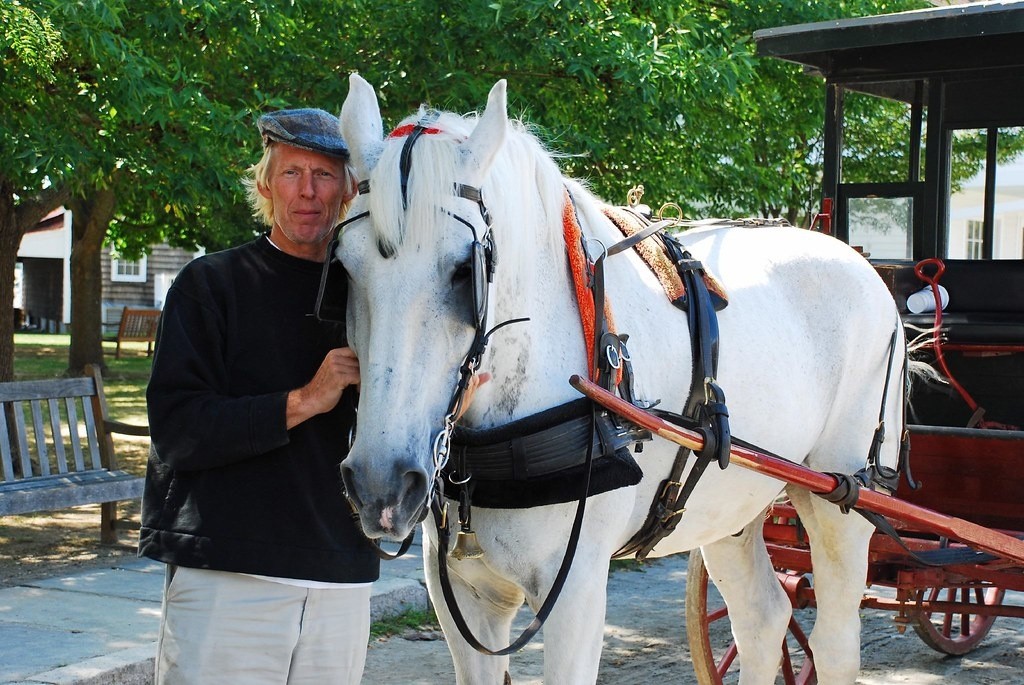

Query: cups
[907,284,949,314]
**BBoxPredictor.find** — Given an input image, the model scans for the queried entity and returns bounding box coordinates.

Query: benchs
[0,364,151,554]
[875,259,1023,356]
[102,306,161,360]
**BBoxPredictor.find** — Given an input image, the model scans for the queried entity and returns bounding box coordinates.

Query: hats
[256,108,350,158]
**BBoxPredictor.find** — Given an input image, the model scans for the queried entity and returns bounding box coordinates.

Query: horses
[336,71,950,685]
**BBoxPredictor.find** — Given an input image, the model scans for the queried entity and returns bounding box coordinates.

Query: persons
[137,109,380,685]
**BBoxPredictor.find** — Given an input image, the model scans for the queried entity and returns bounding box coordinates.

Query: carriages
[329,1,1024,685]
